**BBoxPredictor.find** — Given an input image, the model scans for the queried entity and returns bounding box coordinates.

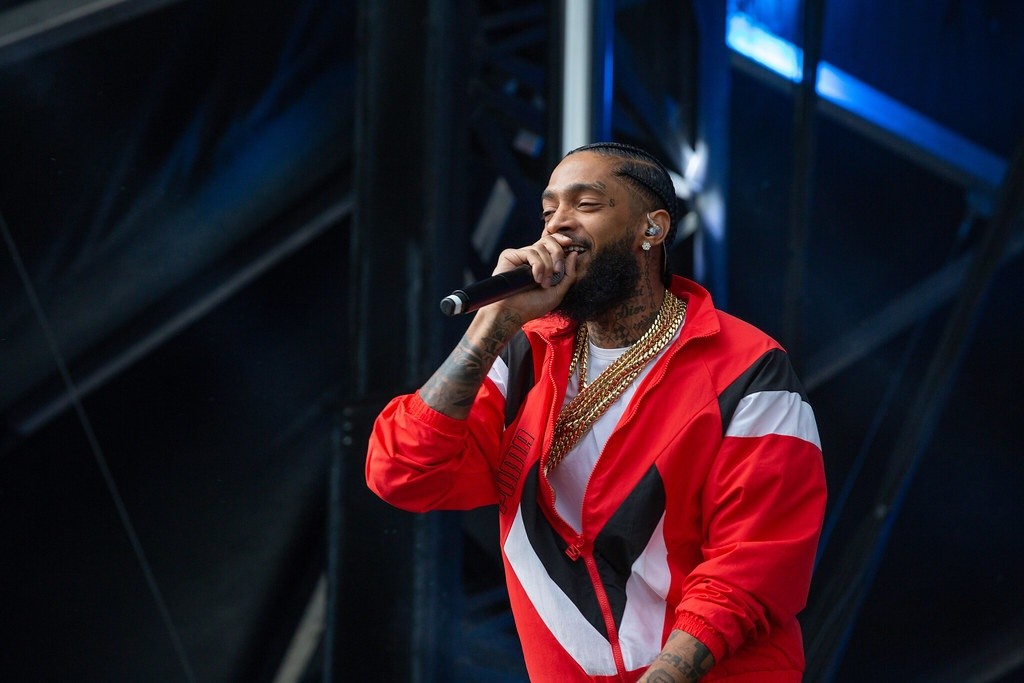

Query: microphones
[440,257,565,317]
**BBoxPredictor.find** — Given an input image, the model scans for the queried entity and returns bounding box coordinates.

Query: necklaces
[544,289,688,478]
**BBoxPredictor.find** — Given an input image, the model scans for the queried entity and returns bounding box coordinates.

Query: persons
[364,143,828,683]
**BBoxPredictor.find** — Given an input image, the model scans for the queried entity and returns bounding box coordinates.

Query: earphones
[646,225,659,237]
